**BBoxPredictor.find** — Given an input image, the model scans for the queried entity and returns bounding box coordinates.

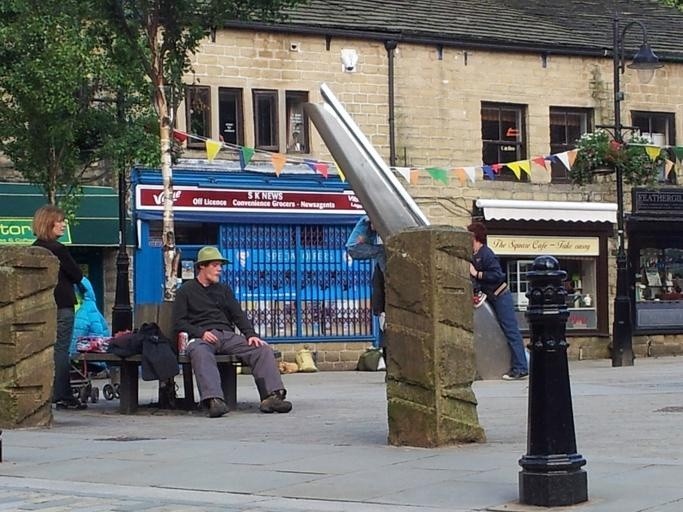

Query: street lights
[592,11,664,368]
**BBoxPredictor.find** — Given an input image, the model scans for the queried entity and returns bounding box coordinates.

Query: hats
[191,244,231,267]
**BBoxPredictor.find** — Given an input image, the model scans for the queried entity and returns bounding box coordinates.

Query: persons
[468,222,530,381]
[172,246,292,417]
[371,263,387,382]
[31,204,111,410]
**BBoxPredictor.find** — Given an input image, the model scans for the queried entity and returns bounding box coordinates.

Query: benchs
[68,302,281,416]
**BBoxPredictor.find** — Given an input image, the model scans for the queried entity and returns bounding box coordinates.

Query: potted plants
[568,130,617,186]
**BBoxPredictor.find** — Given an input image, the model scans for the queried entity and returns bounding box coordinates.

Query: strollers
[68,275,121,400]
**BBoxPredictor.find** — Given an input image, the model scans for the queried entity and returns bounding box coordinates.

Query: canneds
[178,331,189,356]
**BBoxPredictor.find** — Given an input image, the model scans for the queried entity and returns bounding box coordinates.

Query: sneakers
[502,366,530,380]
[55,397,89,412]
[208,397,231,418]
[260,390,293,415]
[473,290,488,310]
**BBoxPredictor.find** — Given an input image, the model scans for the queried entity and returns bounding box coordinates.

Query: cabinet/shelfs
[506,259,534,310]
